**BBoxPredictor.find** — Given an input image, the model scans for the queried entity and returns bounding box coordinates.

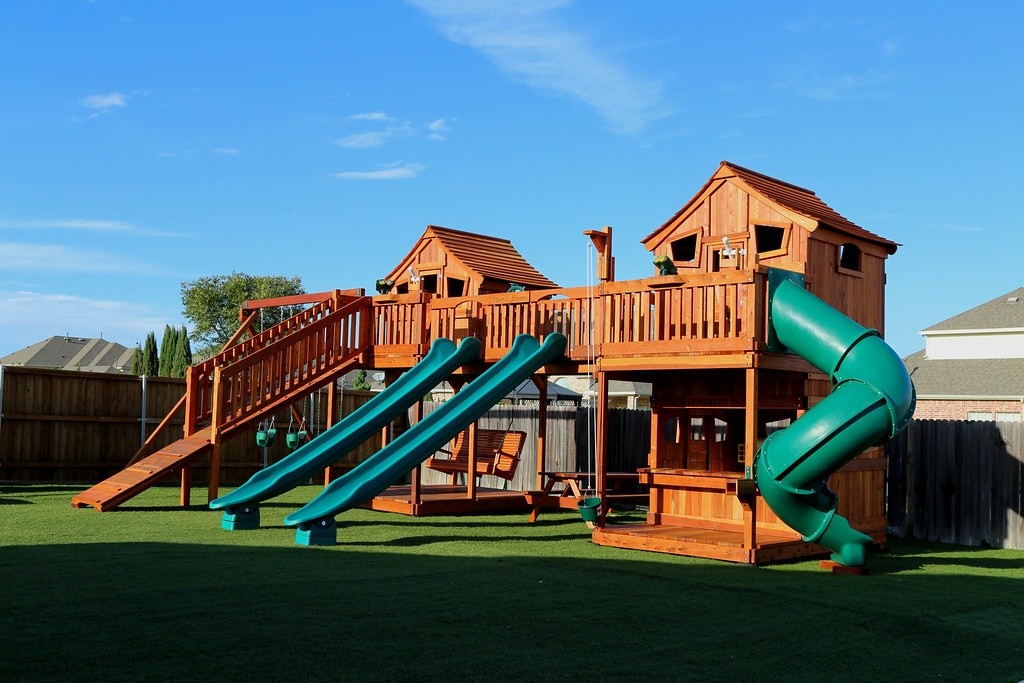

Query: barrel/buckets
[578,488,603,521]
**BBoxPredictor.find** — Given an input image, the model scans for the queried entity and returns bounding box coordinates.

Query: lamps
[653,256,678,276]
[376,279,395,294]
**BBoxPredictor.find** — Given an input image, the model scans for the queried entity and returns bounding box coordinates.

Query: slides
[276,325,570,537]
[200,332,490,515]
[749,268,923,573]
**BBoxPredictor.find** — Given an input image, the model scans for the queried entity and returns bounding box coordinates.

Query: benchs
[578,494,650,502]
[521,489,615,495]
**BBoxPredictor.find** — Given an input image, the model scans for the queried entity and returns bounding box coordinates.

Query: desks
[529,471,639,530]
